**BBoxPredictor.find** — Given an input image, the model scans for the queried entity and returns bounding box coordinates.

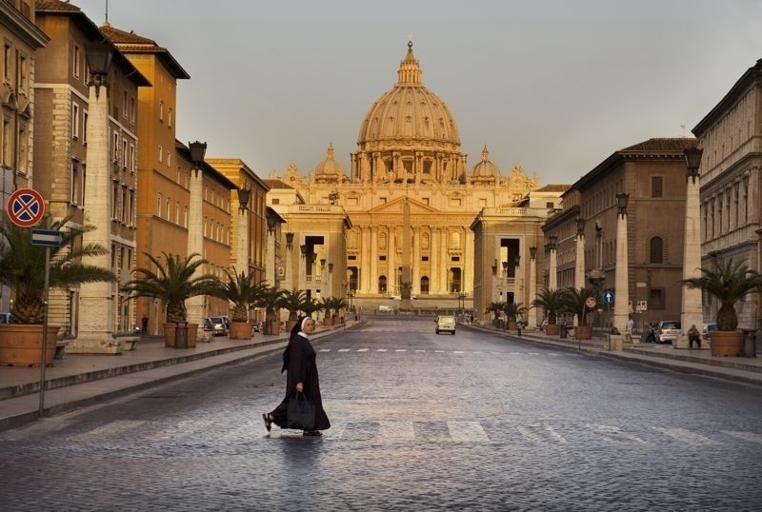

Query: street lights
[185,140,214,342]
[490,217,586,332]
[63,40,126,354]
[671,145,710,350]
[236,186,334,324]
[604,190,633,342]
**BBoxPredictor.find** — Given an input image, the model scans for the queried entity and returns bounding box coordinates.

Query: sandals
[304,430,323,436]
[263,412,272,431]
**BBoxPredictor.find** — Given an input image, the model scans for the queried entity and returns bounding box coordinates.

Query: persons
[262,315,331,437]
[141,314,149,333]
[645,322,664,343]
[626,314,634,334]
[687,324,702,348]
[470,314,473,323]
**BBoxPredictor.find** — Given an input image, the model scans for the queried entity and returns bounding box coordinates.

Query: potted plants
[529,285,608,340]
[120,247,229,349]
[485,301,529,330]
[672,252,762,357]
[0,202,118,370]
[222,262,348,340]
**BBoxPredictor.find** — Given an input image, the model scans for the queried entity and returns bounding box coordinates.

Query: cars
[434,315,456,335]
[656,320,718,344]
[204,315,232,336]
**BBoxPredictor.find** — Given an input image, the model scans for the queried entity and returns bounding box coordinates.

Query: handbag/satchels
[286,399,316,429]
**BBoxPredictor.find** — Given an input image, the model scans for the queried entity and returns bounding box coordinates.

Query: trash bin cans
[175,321,189,349]
[560,325,567,338]
[265,319,272,335]
[742,334,756,358]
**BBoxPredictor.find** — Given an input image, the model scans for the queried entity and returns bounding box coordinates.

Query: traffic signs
[30,230,63,248]
